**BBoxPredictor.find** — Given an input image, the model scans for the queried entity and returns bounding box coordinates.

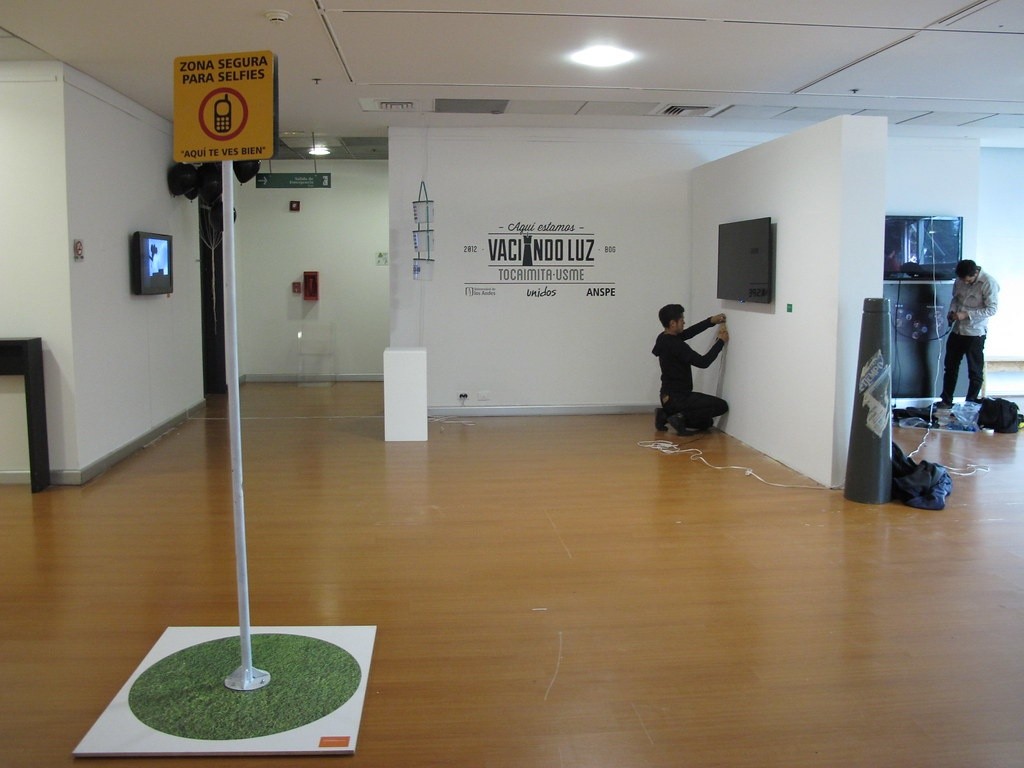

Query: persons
[934,260,1000,408]
[652,303,729,436]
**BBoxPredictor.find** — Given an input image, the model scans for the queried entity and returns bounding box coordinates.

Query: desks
[0,338,49,493]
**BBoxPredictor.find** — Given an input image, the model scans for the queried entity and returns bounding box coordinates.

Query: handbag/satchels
[977,399,1019,434]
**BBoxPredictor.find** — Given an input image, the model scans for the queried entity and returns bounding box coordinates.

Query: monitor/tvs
[717,217,772,304]
[133,231,174,296]
[884,215,963,280]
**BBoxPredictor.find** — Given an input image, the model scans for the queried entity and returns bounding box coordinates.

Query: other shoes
[655,407,668,431]
[668,416,693,436]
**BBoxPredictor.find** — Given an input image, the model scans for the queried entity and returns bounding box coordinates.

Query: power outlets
[458,391,470,400]
